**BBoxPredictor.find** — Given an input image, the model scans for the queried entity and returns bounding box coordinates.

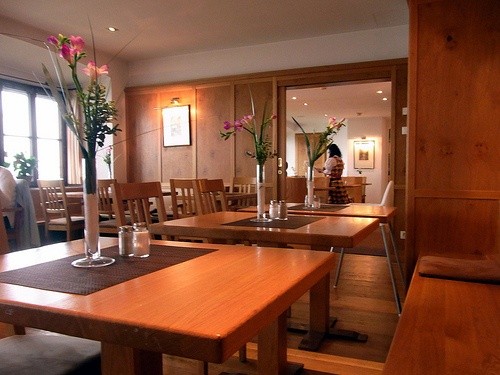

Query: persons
[318,144,352,204]
[0,164,18,232]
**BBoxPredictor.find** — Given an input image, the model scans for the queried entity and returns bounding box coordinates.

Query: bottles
[118,225,135,257]
[278,200,288,221]
[133,222,150,257]
[313,195,320,209]
[269,199,279,220]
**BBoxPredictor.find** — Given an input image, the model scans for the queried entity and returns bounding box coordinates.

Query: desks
[147,210,381,374]
[382,253,499,374]
[237,199,398,352]
[175,191,258,222]
[47,190,115,233]
[98,187,178,220]
[1,235,337,375]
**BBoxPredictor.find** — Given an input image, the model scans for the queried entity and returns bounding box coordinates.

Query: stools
[324,181,406,316]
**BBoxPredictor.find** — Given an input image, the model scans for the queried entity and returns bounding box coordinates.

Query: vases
[105,164,113,180]
[16,175,33,187]
[303,162,317,209]
[251,164,271,222]
[70,152,116,268]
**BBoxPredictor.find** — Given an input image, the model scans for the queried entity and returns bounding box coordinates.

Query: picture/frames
[161,104,193,148]
[352,139,376,170]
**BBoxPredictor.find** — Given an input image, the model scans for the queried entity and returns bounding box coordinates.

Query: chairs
[4,179,30,250]
[0,207,103,375]
[36,177,88,242]
[225,175,259,214]
[109,180,172,242]
[191,176,256,247]
[93,177,134,237]
[168,177,210,243]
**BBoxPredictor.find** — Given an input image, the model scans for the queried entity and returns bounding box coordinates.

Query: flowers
[291,114,344,182]
[99,146,119,167]
[31,12,119,183]
[220,84,280,184]
[14,152,38,177]
